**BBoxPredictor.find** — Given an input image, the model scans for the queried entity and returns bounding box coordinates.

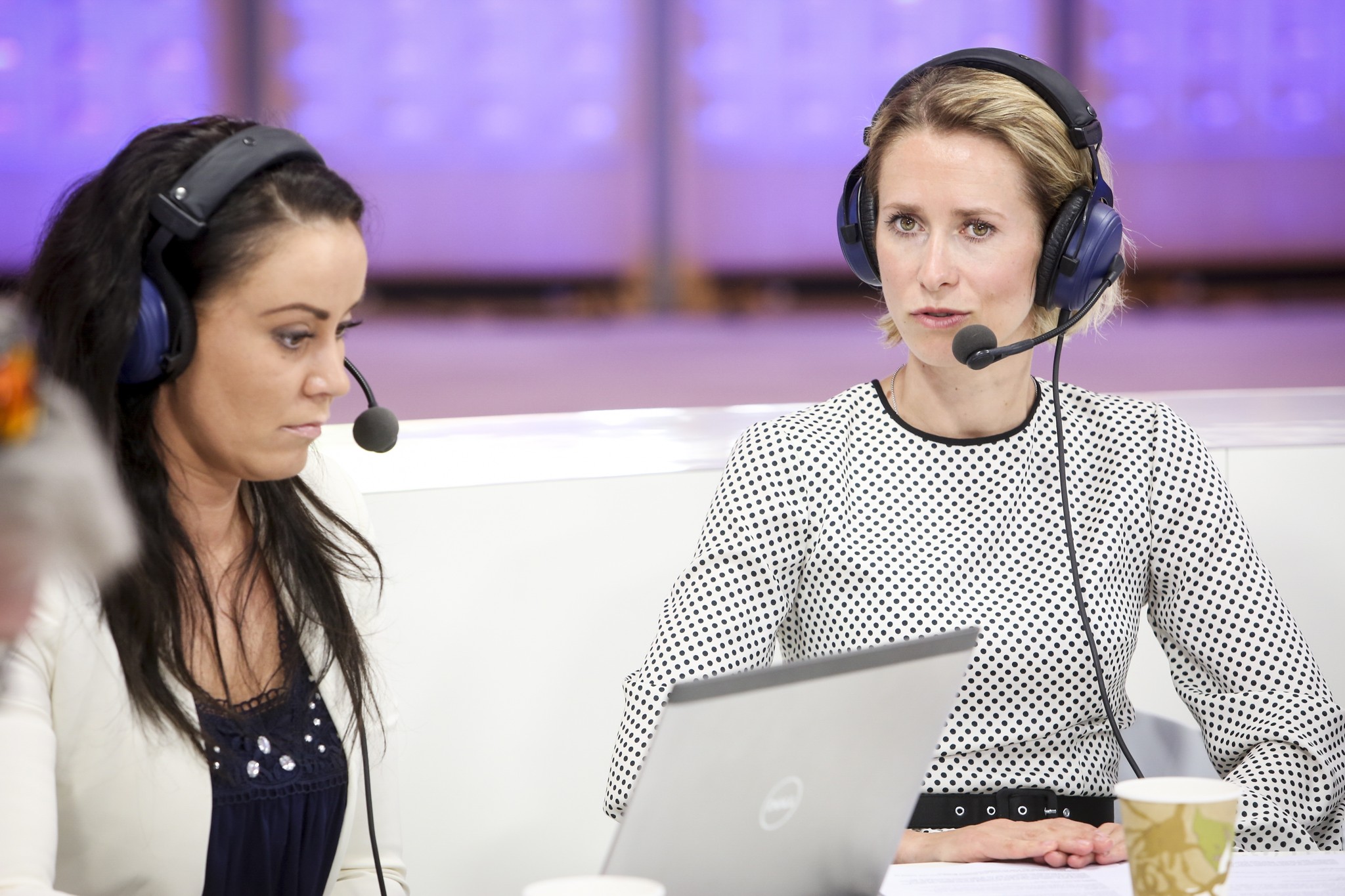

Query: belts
[907,786,1115,828]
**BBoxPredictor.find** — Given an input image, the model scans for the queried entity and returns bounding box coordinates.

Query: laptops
[597,627,979,896]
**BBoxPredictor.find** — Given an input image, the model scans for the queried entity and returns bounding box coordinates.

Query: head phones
[836,48,1125,328]
[114,126,328,393]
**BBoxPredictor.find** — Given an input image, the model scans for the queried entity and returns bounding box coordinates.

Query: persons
[604,46,1345,870]
[0,114,409,896]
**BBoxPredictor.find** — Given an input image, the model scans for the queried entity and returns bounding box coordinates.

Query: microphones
[952,280,1110,370]
[343,357,400,453]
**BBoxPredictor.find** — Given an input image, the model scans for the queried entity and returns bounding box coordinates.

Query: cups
[1113,776,1242,896]
[523,876,666,896]
